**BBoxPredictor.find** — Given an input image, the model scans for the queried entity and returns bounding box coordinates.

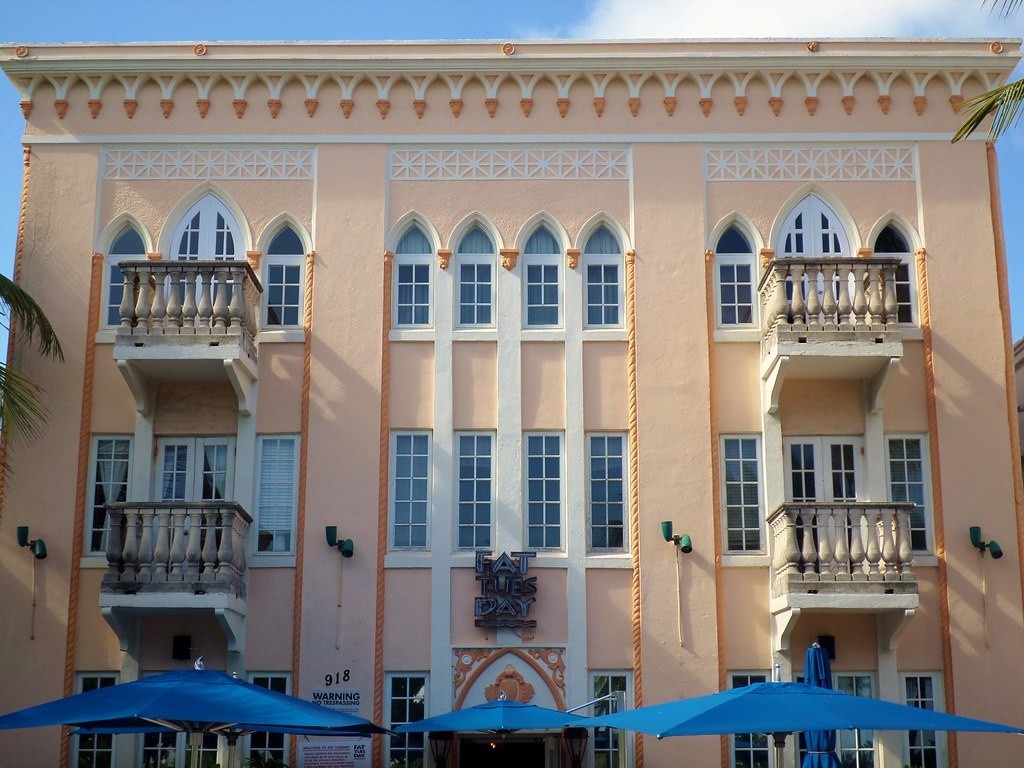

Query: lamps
[16,525,49,560]
[173,635,191,661]
[969,526,1003,561]
[660,520,692,555]
[428,731,455,768]
[326,525,354,558]
[563,728,590,768]
[490,738,496,749]
[496,729,510,739]
[818,634,836,660]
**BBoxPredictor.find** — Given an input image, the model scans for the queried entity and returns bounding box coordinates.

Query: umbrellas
[804,643,843,768]
[564,672,1024,768]
[0,657,395,768]
[392,693,591,768]
